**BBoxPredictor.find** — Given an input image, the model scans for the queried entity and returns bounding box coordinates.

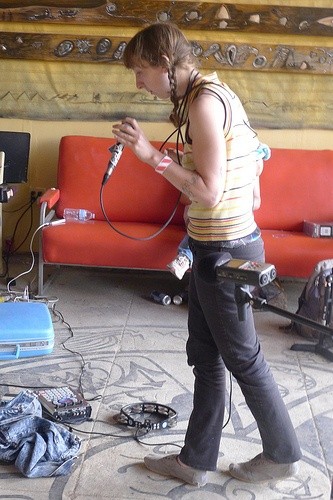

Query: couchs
[37,136,333,299]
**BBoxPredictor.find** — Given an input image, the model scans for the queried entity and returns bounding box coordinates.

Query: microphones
[102,123,134,185]
[196,252,277,322]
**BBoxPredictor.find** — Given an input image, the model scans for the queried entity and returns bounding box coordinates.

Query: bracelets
[154,155,173,176]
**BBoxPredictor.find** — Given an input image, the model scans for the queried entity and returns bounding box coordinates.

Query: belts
[193,227,262,249]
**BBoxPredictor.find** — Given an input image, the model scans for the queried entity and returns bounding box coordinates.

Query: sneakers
[229,453,298,483]
[144,454,206,487]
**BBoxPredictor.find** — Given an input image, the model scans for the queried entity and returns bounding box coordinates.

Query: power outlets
[29,187,46,206]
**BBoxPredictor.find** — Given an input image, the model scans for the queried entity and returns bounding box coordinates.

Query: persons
[166,231,194,280]
[112,24,304,486]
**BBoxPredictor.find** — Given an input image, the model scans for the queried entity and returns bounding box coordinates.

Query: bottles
[63,208,95,221]
